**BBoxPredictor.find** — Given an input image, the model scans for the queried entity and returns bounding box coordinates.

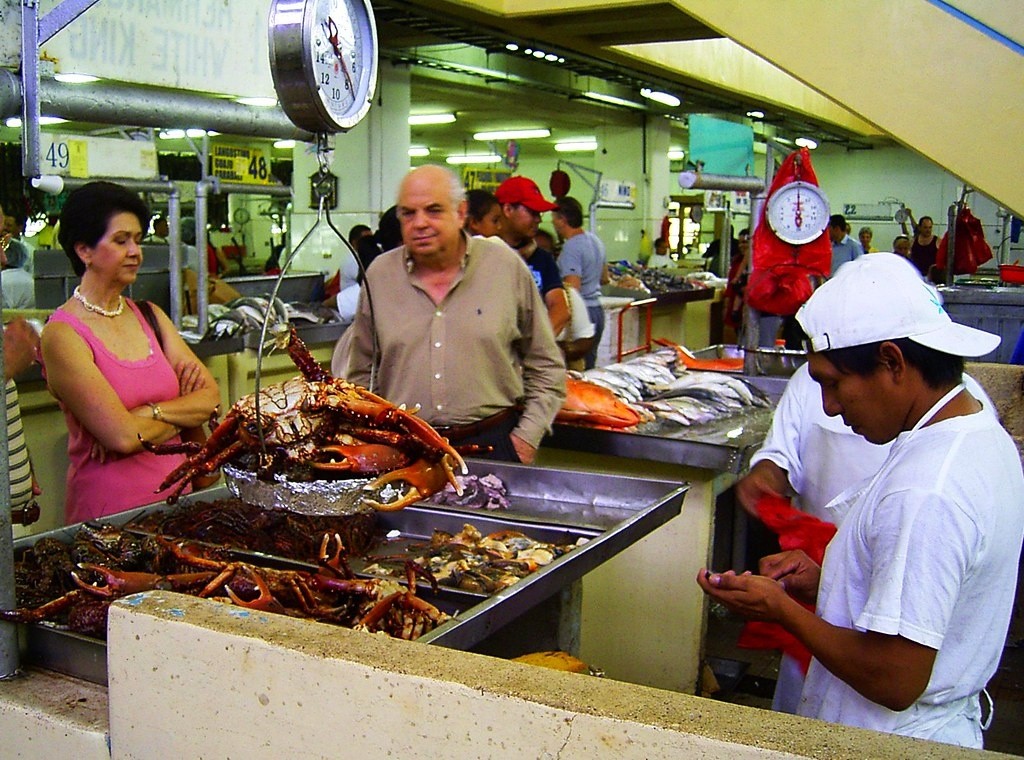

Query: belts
[12,499,41,526]
[442,409,512,444]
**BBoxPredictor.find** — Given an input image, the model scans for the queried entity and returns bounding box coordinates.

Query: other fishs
[567,336,774,426]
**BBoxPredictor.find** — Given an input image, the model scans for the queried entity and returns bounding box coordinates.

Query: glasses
[0,233,12,251]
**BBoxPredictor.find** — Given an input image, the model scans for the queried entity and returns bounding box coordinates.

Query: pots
[738,346,808,376]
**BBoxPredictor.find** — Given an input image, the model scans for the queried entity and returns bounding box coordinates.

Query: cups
[32,176,63,195]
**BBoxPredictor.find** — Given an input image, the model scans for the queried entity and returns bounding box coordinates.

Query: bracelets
[144,402,164,421]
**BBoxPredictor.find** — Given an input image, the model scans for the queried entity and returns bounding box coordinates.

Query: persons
[698,246,1024,750]
[551,197,610,370]
[40,182,223,530]
[820,214,864,283]
[646,236,689,268]
[703,222,750,344]
[462,188,503,239]
[907,216,944,277]
[0,208,39,534]
[179,217,218,275]
[532,227,555,255]
[494,174,572,343]
[143,216,169,244]
[345,162,568,468]
[733,347,1002,717]
[892,234,912,262]
[857,226,879,254]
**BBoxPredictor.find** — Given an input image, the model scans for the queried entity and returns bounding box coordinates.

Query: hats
[794,254,1002,356]
[495,176,559,213]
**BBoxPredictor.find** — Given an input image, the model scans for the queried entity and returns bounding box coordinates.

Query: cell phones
[705,571,714,580]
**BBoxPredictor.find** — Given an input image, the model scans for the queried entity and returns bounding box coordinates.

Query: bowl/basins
[998,264,1024,285]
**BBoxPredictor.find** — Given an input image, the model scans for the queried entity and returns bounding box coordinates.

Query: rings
[33,346,37,350]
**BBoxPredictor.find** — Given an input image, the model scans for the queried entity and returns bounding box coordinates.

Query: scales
[736,132,830,379]
[220,1,423,516]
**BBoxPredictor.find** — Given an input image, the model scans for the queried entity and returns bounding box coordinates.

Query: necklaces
[74,284,125,316]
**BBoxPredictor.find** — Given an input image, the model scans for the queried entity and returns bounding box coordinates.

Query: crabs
[0,472,591,641]
[551,379,640,433]
[133,320,470,512]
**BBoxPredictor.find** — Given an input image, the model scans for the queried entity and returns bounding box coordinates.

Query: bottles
[773,339,787,352]
